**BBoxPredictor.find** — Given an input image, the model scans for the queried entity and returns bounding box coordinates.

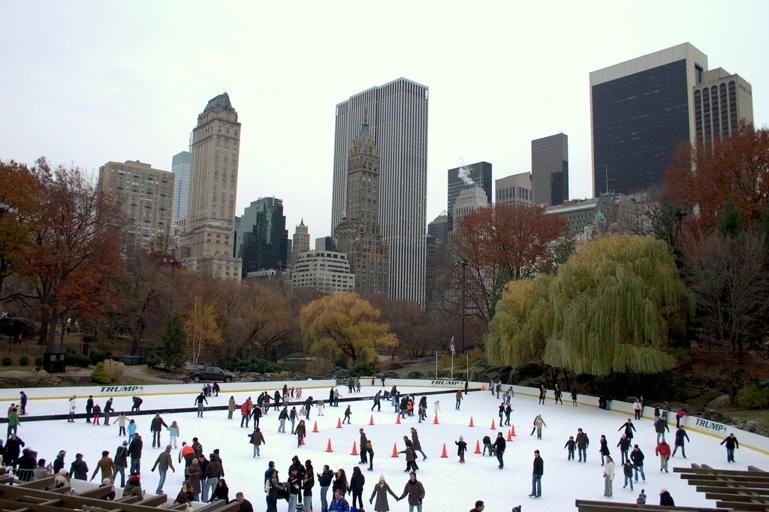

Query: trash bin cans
[45,352,65,372]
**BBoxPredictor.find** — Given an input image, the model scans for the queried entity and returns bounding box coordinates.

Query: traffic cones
[396,414,401,424]
[325,437,334,453]
[312,420,319,432]
[433,413,439,424]
[480,385,486,391]
[467,416,475,427]
[336,416,343,428]
[300,434,305,445]
[440,442,448,458]
[505,429,512,441]
[510,424,517,436]
[368,414,374,425]
[391,442,399,457]
[490,417,496,430]
[350,441,359,455]
[474,439,481,453]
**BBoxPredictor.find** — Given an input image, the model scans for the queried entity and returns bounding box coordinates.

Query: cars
[0,317,40,339]
[666,316,728,344]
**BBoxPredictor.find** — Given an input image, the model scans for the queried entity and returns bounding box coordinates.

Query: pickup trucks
[183,366,235,382]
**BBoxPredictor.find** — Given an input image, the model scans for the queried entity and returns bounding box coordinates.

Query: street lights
[287,468,312,512]
[455,260,471,354]
[160,257,188,366]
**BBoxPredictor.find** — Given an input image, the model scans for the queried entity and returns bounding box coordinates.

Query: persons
[371,375,427,423]
[194,382,221,417]
[150,413,229,504]
[264,456,365,511]
[488,377,514,427]
[720,433,739,462]
[565,428,589,463]
[347,378,360,393]
[229,385,352,458]
[598,393,689,506]
[483,432,506,470]
[529,450,543,497]
[370,472,425,511]
[528,415,547,440]
[0,391,144,500]
[398,427,427,472]
[456,381,468,410]
[230,492,253,512]
[472,501,485,511]
[358,429,375,470]
[455,435,467,463]
[538,383,579,405]
